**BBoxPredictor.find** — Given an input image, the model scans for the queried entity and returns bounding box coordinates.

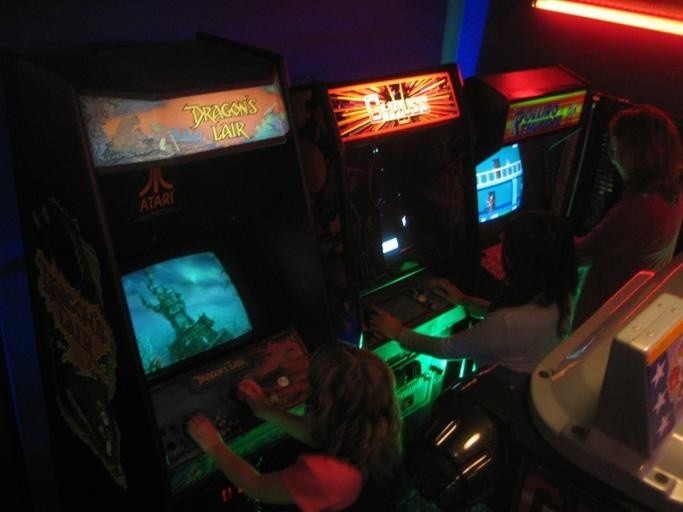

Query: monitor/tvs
[120,249,254,375]
[348,190,414,294]
[476,141,524,223]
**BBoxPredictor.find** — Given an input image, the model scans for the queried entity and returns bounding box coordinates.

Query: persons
[368,212,570,375]
[186,338,403,512]
[573,103,683,331]
